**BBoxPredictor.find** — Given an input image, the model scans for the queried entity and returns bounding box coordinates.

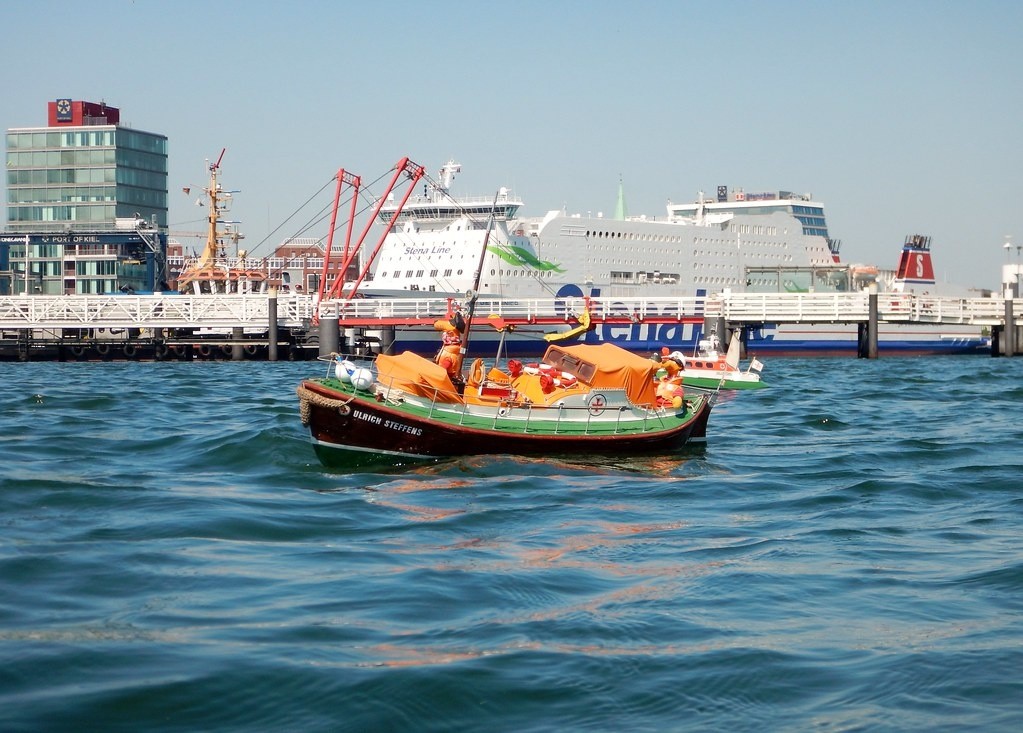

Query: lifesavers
[96,345,110,355]
[200,345,211,356]
[153,345,169,357]
[245,345,257,355]
[174,345,187,357]
[70,346,85,356]
[522,362,579,389]
[222,345,233,357]
[124,346,137,357]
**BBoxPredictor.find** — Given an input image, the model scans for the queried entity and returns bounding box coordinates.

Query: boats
[309,159,994,358]
[295,189,727,470]
[661,325,771,391]
